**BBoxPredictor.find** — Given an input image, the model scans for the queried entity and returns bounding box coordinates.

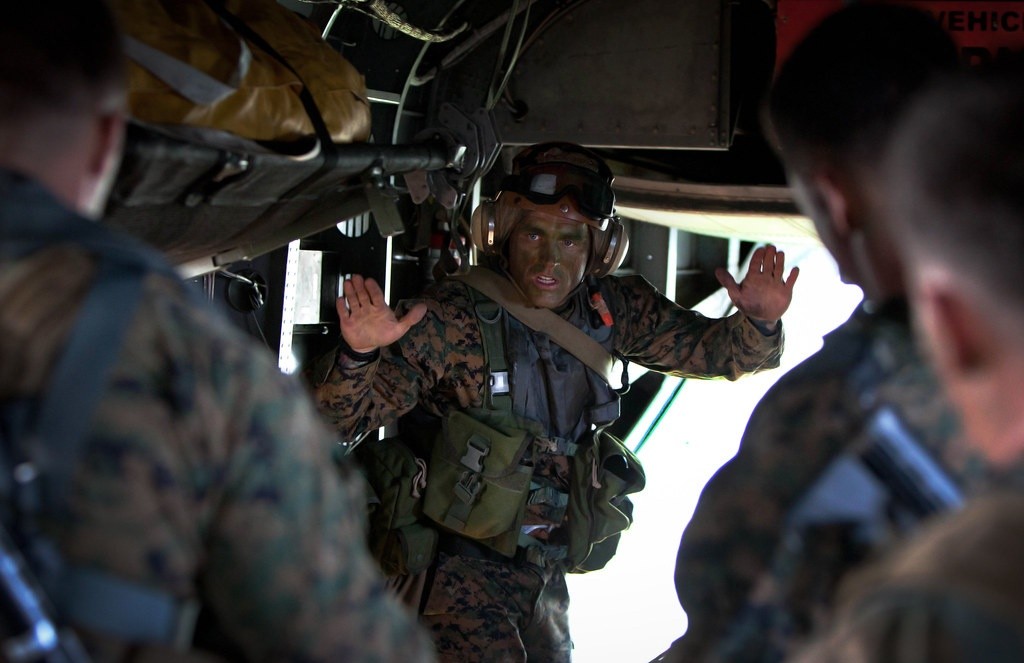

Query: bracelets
[338,335,380,362]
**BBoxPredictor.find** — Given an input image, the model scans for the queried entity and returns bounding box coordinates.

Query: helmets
[493,141,615,231]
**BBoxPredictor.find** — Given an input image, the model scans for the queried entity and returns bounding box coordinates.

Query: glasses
[514,161,615,222]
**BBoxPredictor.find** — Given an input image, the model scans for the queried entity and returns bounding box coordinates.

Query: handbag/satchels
[106,0,374,149]
[420,409,540,539]
[566,429,645,574]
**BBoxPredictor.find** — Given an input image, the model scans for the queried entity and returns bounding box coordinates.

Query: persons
[293,142,798,663]
[0,0,436,663]
[790,46,1024,663]
[651,0,968,663]
